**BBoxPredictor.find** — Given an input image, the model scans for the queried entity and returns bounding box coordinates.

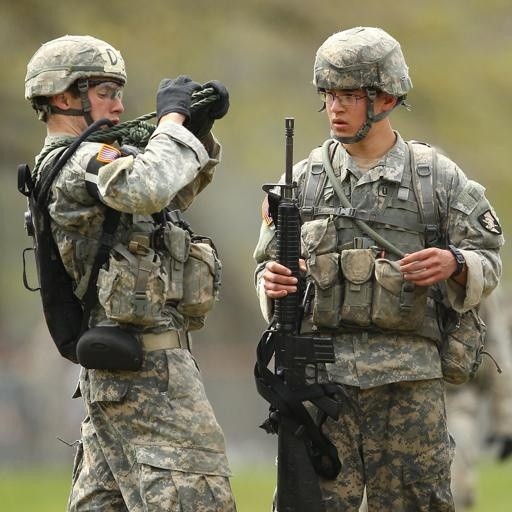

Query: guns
[264,118,345,512]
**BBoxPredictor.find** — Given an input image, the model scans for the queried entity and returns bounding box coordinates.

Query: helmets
[312,26,413,98]
[24,36,127,98]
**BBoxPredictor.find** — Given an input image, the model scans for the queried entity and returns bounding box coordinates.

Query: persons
[252,26,506,512]
[19,34,236,512]
[358,289,512,510]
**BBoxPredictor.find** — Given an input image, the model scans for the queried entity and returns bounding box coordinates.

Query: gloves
[203,80,229,118]
[157,75,201,124]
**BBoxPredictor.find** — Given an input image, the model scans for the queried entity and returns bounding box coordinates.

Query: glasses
[95,84,124,104]
[317,91,370,105]
